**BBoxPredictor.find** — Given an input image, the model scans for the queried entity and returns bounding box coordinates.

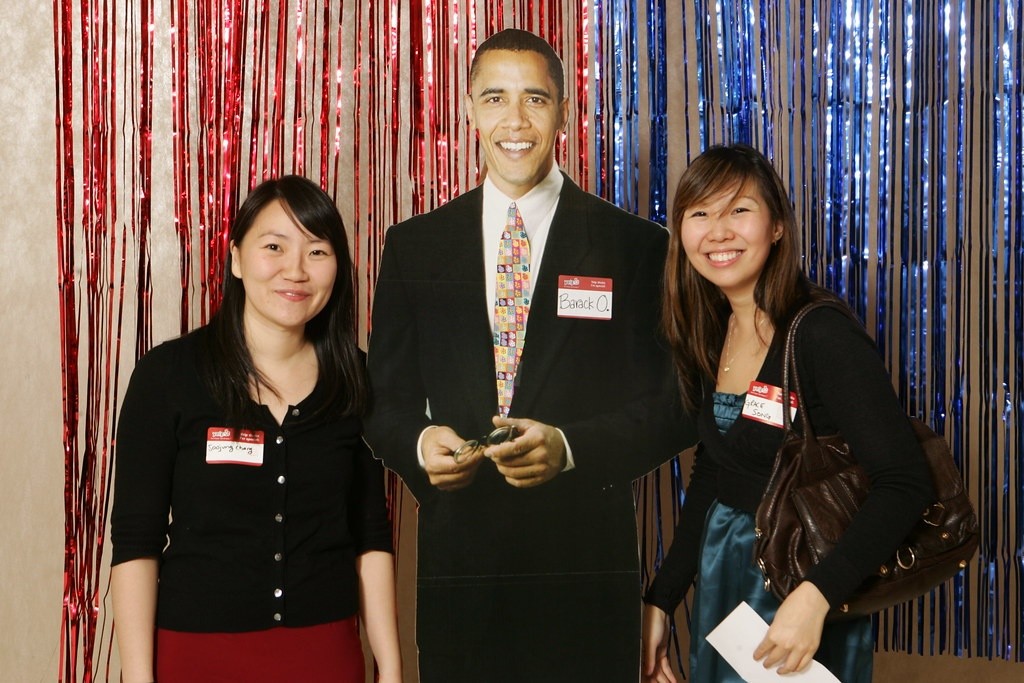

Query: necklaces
[724,312,766,373]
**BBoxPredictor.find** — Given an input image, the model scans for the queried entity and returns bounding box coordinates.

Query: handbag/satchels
[755,302,978,620]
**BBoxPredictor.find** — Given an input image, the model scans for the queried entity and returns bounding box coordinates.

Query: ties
[492,201,533,418]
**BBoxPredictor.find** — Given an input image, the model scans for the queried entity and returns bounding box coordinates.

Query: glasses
[454,425,518,463]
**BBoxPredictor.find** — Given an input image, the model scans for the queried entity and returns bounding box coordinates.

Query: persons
[354,28,705,683]
[113,175,408,683]
[643,144,938,683]
